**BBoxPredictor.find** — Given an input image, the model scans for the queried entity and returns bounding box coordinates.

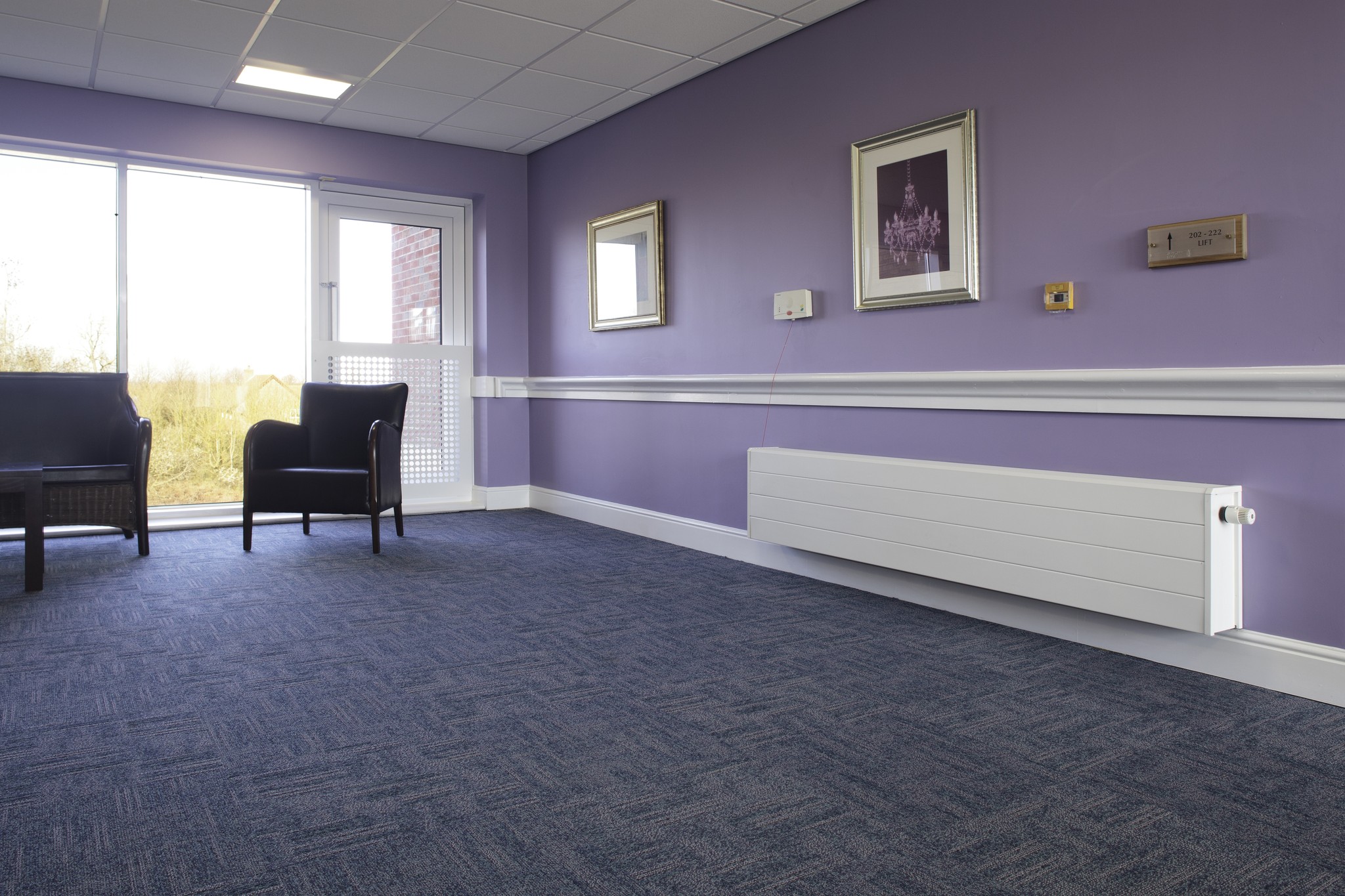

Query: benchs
[0,371,152,555]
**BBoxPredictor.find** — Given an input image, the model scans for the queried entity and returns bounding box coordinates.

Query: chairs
[243,382,409,554]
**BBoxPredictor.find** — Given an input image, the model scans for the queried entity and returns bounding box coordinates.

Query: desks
[0,463,45,591]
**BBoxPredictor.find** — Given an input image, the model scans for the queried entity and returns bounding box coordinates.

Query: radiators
[746,446,1255,637]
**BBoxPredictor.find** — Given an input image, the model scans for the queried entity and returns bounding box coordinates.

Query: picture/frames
[851,108,979,311]
[587,200,667,332]
